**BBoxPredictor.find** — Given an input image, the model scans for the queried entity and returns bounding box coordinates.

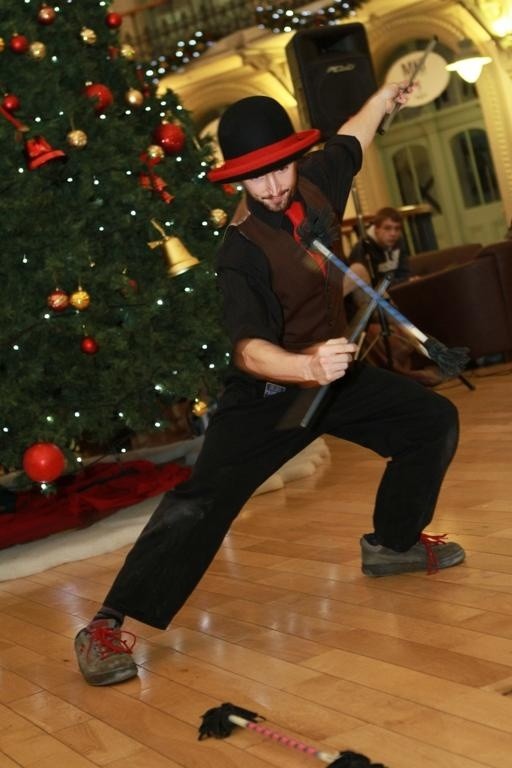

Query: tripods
[348,177,476,393]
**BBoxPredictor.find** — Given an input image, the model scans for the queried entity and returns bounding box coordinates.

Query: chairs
[386,241,512,363]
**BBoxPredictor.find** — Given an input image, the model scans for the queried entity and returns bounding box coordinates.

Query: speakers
[284,21,379,147]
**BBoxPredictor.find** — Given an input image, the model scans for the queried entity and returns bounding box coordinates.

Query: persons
[343,203,414,373]
[70,74,468,686]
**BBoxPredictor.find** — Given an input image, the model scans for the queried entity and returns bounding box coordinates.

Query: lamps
[445,38,494,84]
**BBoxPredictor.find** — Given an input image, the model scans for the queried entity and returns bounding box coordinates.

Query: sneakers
[74,619,137,686]
[360,534,464,576]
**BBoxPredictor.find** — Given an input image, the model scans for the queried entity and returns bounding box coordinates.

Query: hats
[207,96,320,184]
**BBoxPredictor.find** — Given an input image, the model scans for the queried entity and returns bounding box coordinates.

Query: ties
[285,201,327,276]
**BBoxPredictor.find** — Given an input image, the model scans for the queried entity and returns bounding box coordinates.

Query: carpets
[0,433,330,582]
